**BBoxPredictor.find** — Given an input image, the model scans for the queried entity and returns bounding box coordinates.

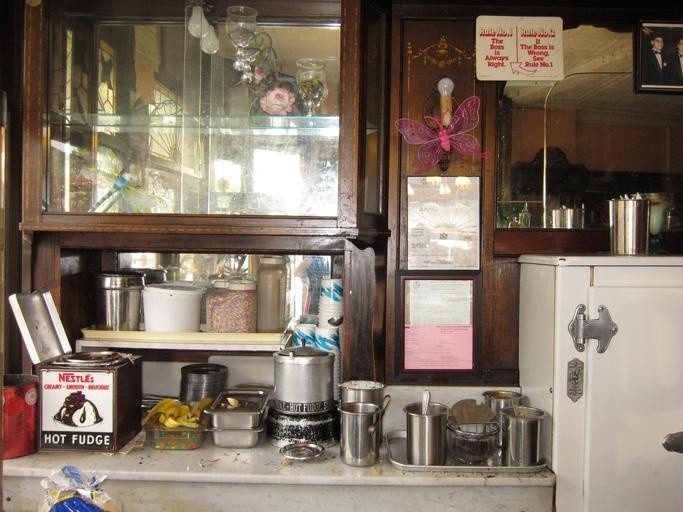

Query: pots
[272,337,335,416]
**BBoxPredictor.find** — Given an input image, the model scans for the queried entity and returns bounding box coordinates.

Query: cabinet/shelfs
[17,1,391,241]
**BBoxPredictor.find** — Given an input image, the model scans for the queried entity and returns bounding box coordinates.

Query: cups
[482,389,524,410]
[339,379,386,410]
[294,279,342,402]
[606,198,653,257]
[501,407,546,465]
[401,401,448,467]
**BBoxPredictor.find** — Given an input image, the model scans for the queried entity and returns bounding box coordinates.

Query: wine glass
[224,5,328,117]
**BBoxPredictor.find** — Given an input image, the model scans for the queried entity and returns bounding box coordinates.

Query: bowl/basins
[444,422,500,464]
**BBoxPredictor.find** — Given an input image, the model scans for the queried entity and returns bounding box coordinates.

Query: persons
[643,34,667,84]
[664,38,681,85]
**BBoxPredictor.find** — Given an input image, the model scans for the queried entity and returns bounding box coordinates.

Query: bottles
[257,255,286,332]
[93,269,143,333]
[205,276,258,332]
[518,201,530,228]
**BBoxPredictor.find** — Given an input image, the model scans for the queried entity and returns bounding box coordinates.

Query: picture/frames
[392,268,483,383]
[631,16,683,96]
